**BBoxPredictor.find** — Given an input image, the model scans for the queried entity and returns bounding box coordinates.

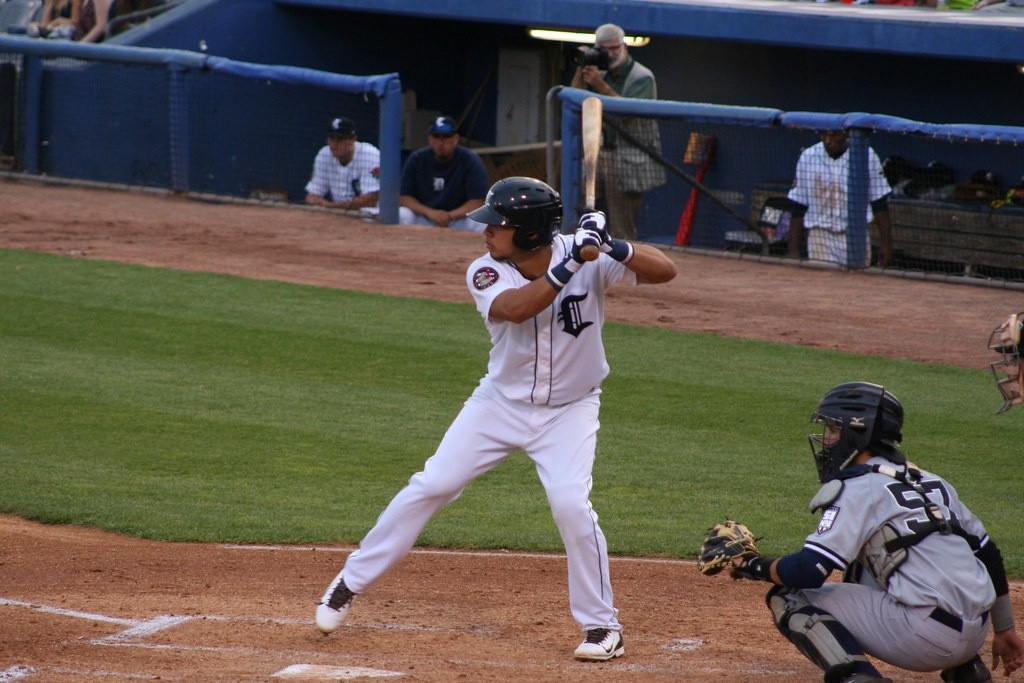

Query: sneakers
[315,568,360,633]
[574,628,625,661]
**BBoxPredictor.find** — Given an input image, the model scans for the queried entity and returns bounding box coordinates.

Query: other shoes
[941,654,993,683]
[841,674,891,683]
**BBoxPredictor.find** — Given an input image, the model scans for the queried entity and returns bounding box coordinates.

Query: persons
[303,116,381,223]
[398,116,490,232]
[697,380,1024,683]
[314,177,679,662]
[76,0,167,43]
[39,0,81,39]
[786,129,898,269]
[987,311,1024,414]
[570,23,668,239]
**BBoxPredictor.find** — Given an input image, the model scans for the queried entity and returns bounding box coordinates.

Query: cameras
[573,46,609,70]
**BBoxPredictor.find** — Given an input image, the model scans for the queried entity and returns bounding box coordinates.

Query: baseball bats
[579,95,603,261]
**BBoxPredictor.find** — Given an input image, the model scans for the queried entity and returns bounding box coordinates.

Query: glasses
[432,134,454,139]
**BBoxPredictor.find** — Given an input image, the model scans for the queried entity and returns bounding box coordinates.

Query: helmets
[466,176,562,251]
[814,382,904,483]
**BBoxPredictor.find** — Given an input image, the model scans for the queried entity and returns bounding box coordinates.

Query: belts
[929,606,989,632]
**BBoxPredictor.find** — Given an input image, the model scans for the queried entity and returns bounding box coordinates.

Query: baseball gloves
[697,518,762,576]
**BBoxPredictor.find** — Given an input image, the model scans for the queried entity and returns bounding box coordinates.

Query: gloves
[563,211,614,273]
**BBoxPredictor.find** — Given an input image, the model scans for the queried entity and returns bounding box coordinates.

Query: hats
[328,118,354,138]
[430,116,456,134]
